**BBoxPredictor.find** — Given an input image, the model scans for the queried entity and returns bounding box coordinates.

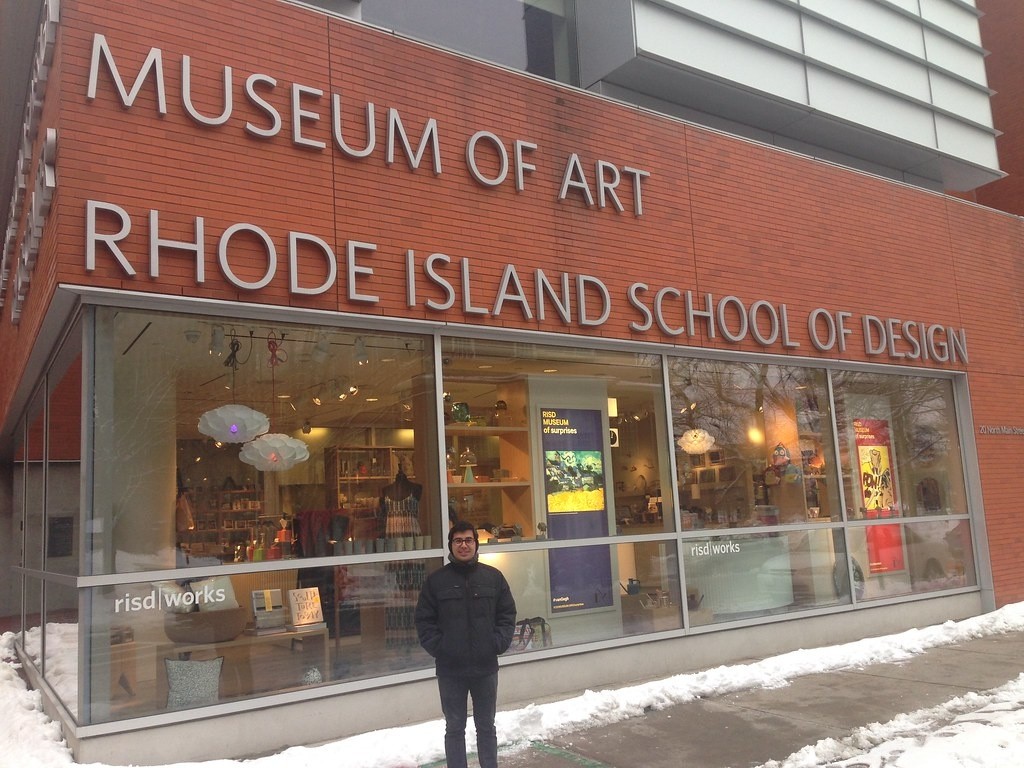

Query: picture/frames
[708,450,725,466]
[718,466,736,482]
[682,470,697,484]
[690,453,705,468]
[699,469,716,484]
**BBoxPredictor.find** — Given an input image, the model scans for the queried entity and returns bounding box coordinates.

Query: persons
[414,520,518,768]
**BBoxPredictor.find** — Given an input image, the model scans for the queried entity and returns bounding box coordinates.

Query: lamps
[442,356,453,369]
[277,376,360,411]
[677,371,716,455]
[310,339,331,363]
[240,328,302,473]
[207,325,225,357]
[353,336,370,365]
[301,418,311,434]
[198,325,270,445]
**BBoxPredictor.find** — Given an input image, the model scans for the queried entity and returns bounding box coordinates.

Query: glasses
[452,538,475,545]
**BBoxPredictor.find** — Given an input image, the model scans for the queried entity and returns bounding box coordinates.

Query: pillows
[114,547,176,596]
[164,656,224,707]
[153,583,194,613]
[190,576,240,612]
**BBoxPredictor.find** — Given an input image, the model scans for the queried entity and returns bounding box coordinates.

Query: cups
[395,538,403,551]
[423,536,431,549]
[354,541,362,554]
[344,542,352,555]
[365,540,373,553]
[405,537,413,550]
[386,539,394,552]
[415,536,423,549]
[376,539,384,553]
[333,544,342,555]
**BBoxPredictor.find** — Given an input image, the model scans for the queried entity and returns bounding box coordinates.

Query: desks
[155,622,330,708]
[110,641,136,702]
[224,555,299,622]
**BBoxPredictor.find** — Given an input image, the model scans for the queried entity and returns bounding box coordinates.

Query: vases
[234,558,244,562]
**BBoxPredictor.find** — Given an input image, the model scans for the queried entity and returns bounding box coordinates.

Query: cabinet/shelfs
[411,373,537,540]
[187,490,261,545]
[762,390,871,604]
[324,446,414,506]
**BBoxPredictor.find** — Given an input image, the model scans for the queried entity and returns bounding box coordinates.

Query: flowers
[234,546,244,559]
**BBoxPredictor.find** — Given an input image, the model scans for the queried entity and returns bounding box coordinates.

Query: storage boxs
[621,586,712,634]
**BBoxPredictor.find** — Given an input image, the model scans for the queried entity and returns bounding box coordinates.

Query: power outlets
[245,326,260,331]
[280,329,291,334]
